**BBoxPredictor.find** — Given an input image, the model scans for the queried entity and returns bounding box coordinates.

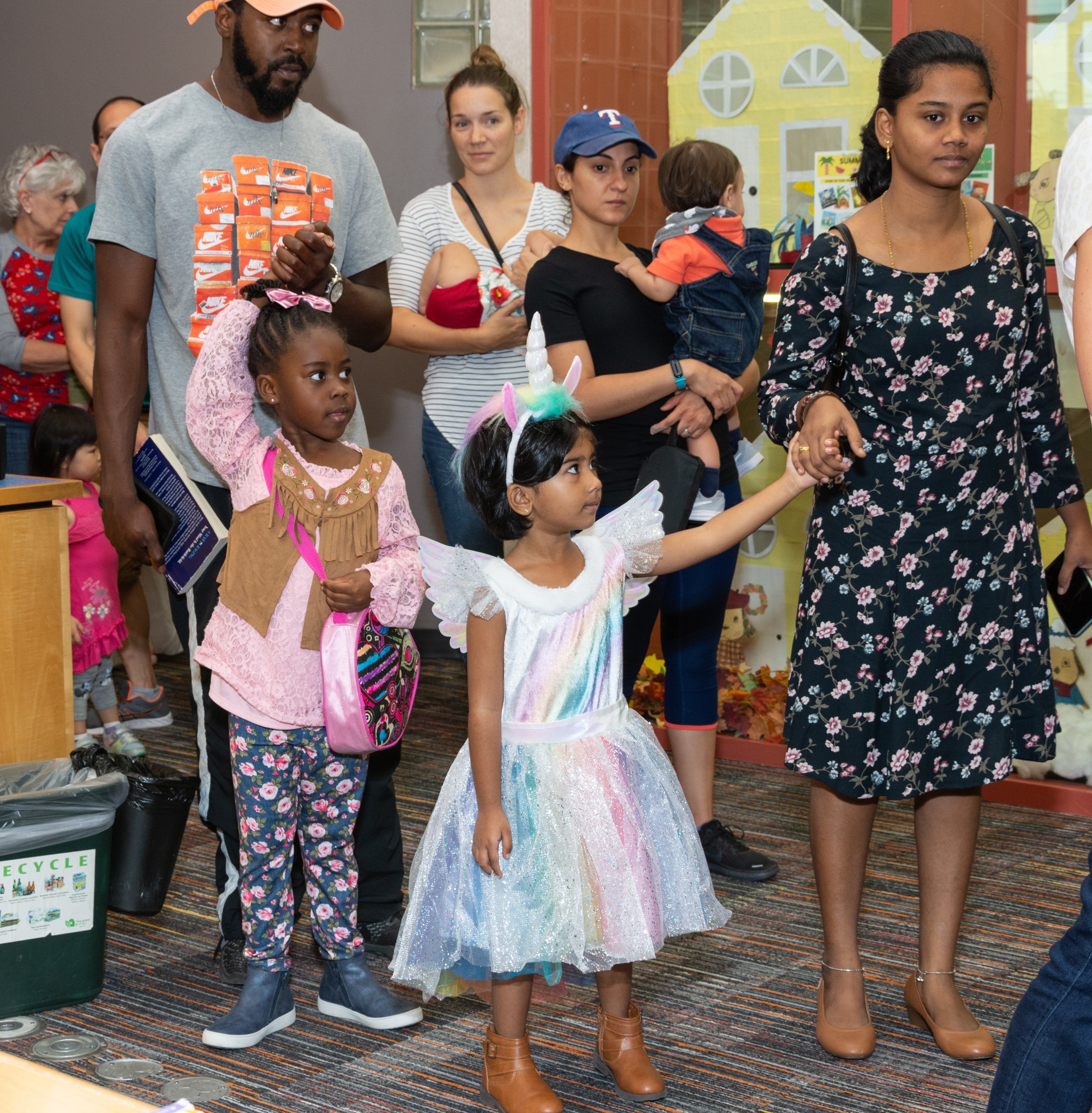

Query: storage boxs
[0,771,128,1020]
[71,744,202,918]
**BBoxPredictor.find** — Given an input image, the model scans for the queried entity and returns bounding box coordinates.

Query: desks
[0,474,83,763]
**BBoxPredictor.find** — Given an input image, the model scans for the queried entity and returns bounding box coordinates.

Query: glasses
[20,149,72,186]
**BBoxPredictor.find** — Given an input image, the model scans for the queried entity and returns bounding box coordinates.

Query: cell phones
[133,478,178,551]
[1045,549,1092,638]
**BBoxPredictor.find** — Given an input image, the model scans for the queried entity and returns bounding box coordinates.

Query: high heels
[902,968,995,1060]
[816,973,877,1060]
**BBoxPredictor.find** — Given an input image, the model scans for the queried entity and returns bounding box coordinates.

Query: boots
[201,962,296,1049]
[593,999,667,1101]
[317,950,424,1030]
[478,1023,564,1113]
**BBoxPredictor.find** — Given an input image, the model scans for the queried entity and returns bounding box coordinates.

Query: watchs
[327,263,344,304]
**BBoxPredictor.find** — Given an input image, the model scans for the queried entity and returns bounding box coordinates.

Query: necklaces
[880,188,974,270]
[212,67,285,204]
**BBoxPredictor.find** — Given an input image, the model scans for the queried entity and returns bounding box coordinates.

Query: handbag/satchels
[319,603,421,756]
[632,422,706,539]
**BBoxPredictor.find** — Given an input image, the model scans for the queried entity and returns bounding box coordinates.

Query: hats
[553,108,658,168]
[187,0,344,31]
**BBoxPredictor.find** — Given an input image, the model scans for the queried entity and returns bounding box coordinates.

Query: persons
[182,223,428,1052]
[614,141,771,522]
[386,45,572,561]
[0,0,1092,1113]
[28,403,146,756]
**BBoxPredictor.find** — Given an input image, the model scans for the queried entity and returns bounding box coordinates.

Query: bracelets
[798,391,840,426]
[703,397,715,416]
[669,360,687,390]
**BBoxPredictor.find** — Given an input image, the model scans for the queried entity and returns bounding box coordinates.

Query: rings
[799,445,810,453]
[684,427,690,437]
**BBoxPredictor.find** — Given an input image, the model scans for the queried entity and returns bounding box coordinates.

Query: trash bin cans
[75,745,199,916]
[1,756,123,1018]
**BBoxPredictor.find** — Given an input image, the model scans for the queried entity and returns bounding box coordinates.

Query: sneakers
[211,935,249,984]
[86,678,174,733]
[74,734,99,749]
[102,723,146,758]
[356,908,407,957]
[694,818,779,883]
[688,486,726,522]
